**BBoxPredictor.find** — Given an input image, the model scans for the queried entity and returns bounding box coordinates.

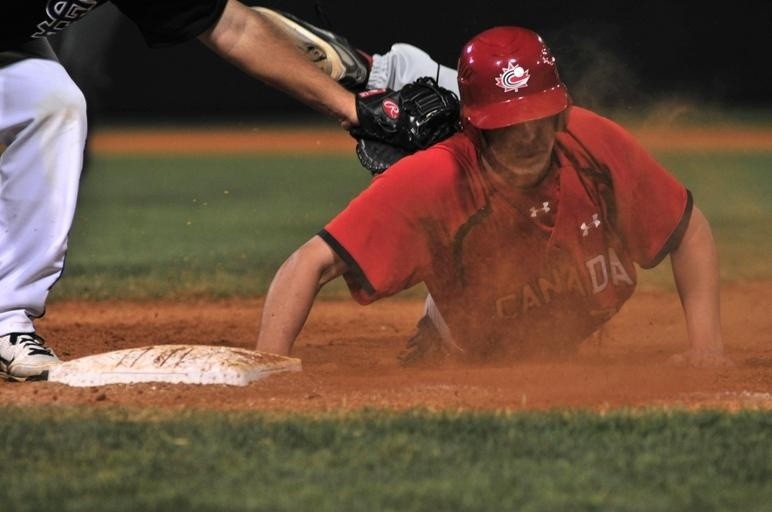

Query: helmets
[458,27,568,130]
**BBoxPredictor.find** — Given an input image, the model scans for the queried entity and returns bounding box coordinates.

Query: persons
[2,0,464,389]
[251,24,744,387]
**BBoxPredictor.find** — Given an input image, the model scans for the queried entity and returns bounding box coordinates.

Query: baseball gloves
[349,76,459,174]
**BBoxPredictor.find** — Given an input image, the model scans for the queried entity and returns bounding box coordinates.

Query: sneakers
[0,333,60,383]
[249,6,372,89]
